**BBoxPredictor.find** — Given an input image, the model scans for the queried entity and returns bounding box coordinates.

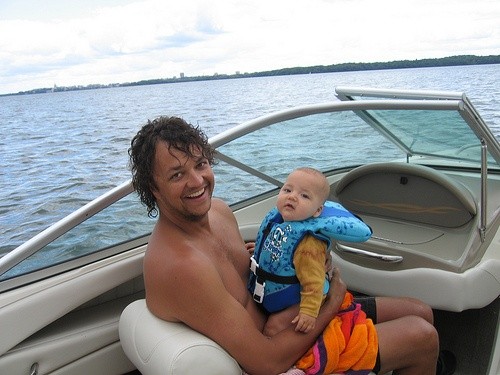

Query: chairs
[116,298,249,375]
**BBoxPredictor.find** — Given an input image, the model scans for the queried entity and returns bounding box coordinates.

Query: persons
[127,115,439,375]
[246,166,335,334]
